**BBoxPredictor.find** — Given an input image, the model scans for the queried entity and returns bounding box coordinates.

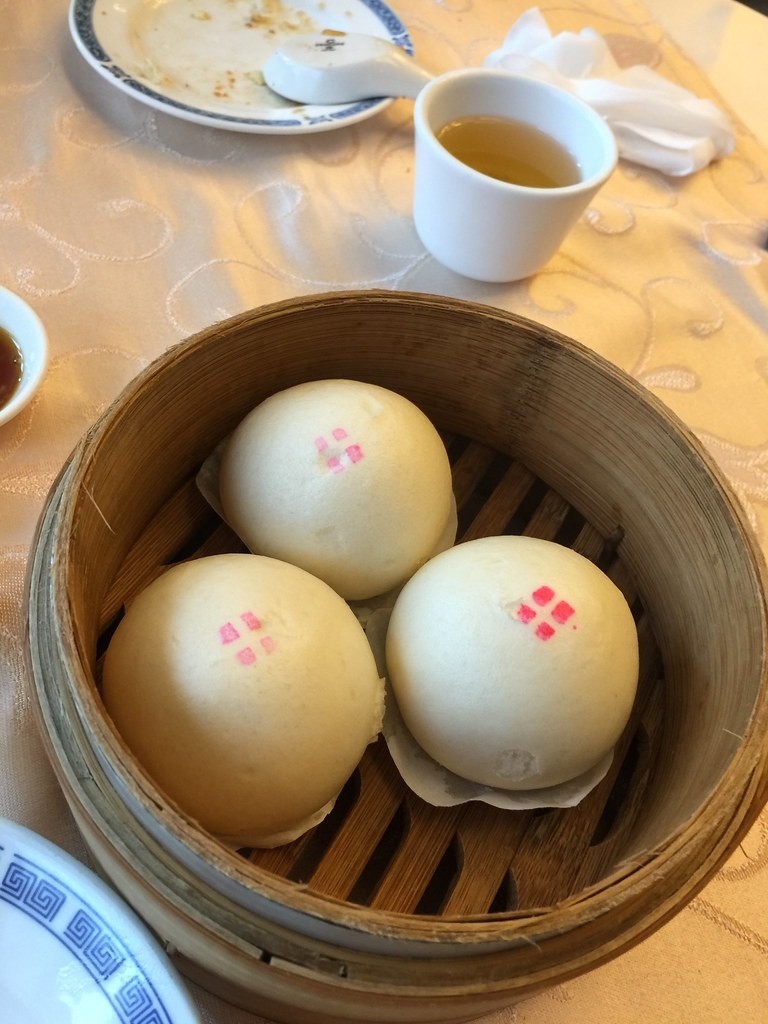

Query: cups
[415,69,618,282]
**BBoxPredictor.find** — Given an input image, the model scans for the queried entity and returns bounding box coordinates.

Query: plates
[68,0,413,133]
[0,817,202,1024]
[0,287,50,427]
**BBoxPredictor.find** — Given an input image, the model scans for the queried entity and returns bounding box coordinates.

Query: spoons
[262,35,437,105]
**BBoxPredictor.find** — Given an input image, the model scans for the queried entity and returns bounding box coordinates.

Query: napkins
[486,8,735,177]
